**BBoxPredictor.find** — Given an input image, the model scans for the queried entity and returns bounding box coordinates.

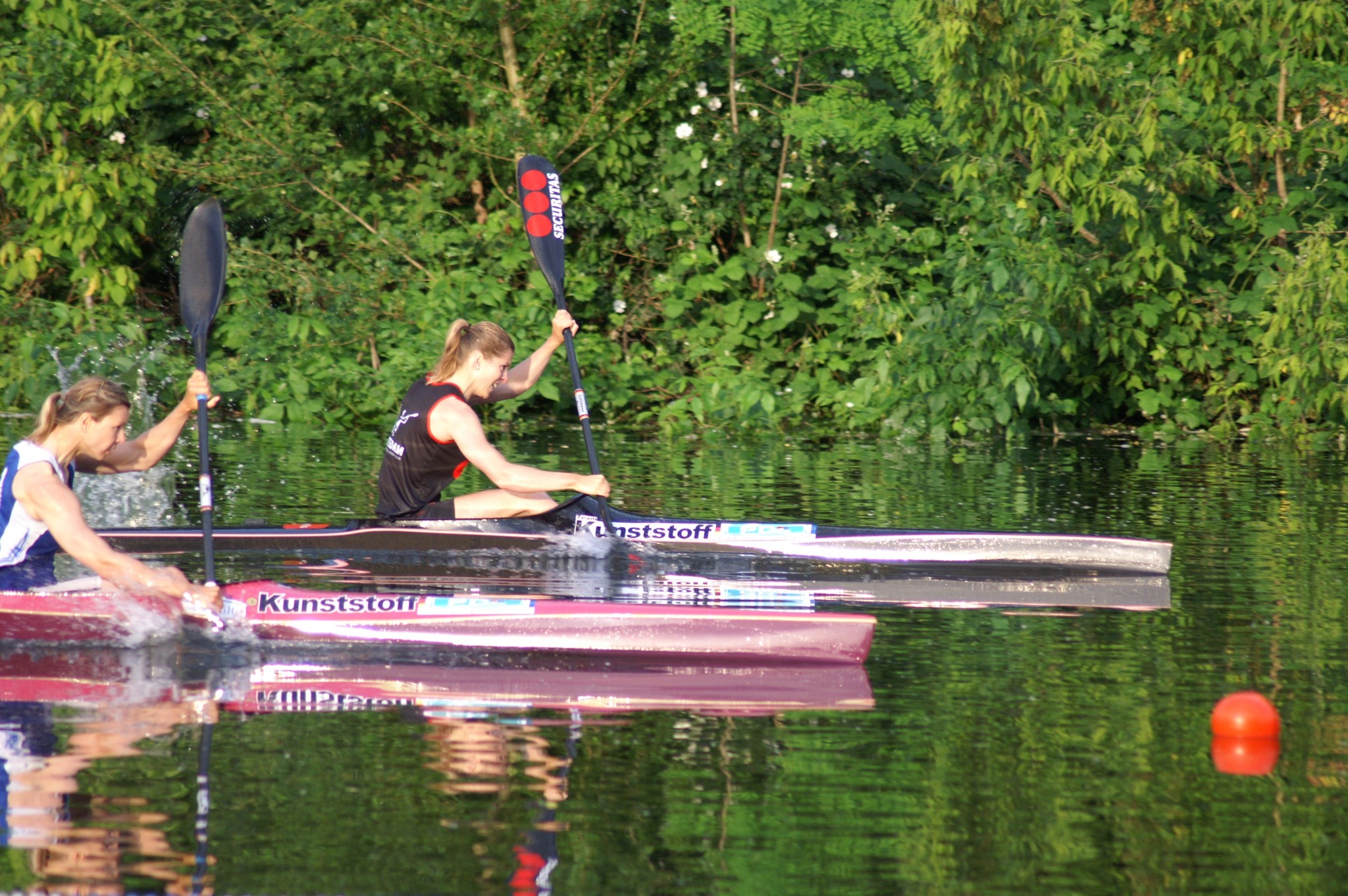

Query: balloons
[1210,689,1282,739]
[1211,739,1281,777]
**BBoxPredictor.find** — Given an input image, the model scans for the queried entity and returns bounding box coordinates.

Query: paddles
[515,153,644,564]
[176,196,229,591]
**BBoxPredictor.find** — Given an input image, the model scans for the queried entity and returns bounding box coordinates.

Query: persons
[0,368,225,617]
[373,309,612,523]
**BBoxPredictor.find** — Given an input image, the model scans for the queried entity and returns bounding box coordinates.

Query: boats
[1,586,880,672]
[89,525,1177,582]
